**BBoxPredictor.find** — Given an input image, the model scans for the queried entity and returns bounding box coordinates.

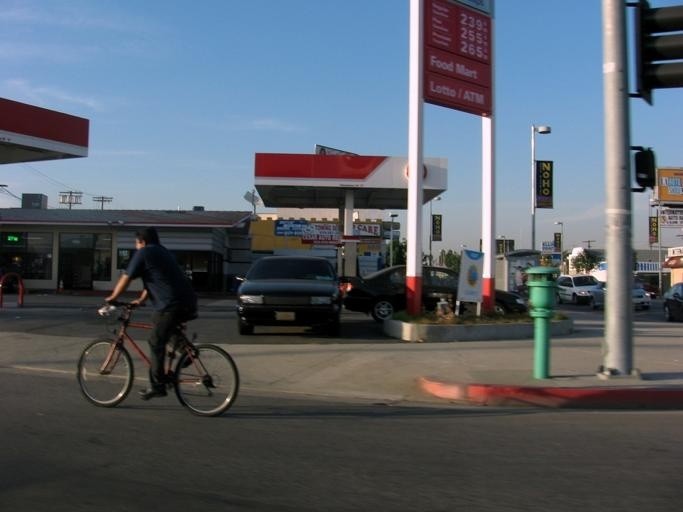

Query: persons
[104,227,197,402]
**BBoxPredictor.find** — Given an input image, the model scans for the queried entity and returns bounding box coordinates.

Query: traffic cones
[57,279,65,295]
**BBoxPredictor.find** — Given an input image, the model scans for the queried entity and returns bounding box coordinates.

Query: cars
[337,264,529,319]
[235,253,345,336]
[553,274,683,323]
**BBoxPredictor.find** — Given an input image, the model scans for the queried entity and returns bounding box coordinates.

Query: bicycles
[74,297,242,417]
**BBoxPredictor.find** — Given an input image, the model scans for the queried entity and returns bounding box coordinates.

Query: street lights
[646,195,656,250]
[554,221,564,275]
[387,212,399,268]
[529,123,556,256]
[429,195,441,267]
[461,244,466,251]
[498,234,506,255]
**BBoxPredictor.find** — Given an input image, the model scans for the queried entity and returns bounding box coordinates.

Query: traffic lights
[632,0,682,106]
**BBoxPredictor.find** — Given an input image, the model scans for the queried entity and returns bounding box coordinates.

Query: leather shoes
[136,387,167,400]
[181,349,200,368]
